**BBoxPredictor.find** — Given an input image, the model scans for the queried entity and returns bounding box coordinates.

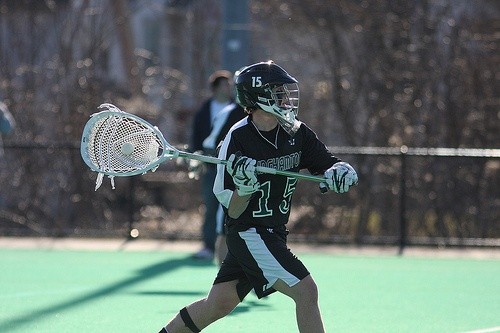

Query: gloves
[227,151,260,196]
[319,161,358,195]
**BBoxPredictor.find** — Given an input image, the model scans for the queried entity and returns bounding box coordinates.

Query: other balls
[121,142,135,156]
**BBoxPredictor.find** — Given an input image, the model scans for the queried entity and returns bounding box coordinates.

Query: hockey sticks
[80,103,326,192]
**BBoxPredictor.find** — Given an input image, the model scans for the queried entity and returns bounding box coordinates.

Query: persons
[155,59,361,333]
[195,98,246,262]
[0,102,16,158]
[187,70,238,258]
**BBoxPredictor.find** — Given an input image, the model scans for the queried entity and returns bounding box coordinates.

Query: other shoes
[191,248,214,259]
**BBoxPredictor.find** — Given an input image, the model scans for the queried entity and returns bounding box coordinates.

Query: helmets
[233,60,300,133]
